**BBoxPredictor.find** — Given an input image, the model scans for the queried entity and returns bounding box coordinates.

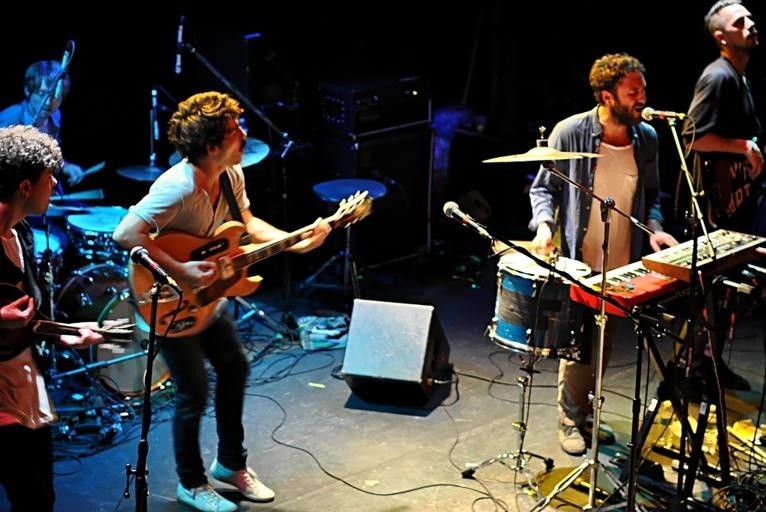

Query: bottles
[658,400,673,450]
[702,404,718,455]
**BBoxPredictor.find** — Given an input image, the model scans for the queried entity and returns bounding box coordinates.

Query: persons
[527,51,680,456]
[658,0,766,403]
[0,59,86,251]
[0,126,106,510]
[112,90,333,512]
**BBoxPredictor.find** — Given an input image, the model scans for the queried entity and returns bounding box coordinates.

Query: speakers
[340,295,451,407]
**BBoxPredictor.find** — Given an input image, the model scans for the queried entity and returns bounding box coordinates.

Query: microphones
[640,106,689,122]
[130,244,180,293]
[171,18,188,78]
[443,200,494,243]
[52,50,71,103]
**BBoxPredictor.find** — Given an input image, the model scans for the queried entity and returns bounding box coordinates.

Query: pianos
[569,230,765,319]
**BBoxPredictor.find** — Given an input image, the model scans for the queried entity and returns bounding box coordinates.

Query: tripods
[532,317,642,511]
[461,373,556,498]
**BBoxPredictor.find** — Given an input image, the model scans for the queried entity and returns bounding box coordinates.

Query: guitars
[129,189,379,339]
[697,136,766,231]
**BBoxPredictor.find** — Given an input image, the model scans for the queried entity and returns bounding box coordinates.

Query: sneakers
[175,479,240,512]
[702,352,754,393]
[557,414,587,455]
[207,456,277,505]
[669,359,721,404]
[580,414,616,445]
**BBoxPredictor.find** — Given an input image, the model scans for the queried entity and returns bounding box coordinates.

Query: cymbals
[168,136,270,168]
[116,166,165,183]
[482,148,605,163]
[238,136,269,169]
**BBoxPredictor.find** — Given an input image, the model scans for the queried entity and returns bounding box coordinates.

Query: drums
[491,252,591,362]
[31,229,66,285]
[67,206,129,266]
[54,269,168,396]
